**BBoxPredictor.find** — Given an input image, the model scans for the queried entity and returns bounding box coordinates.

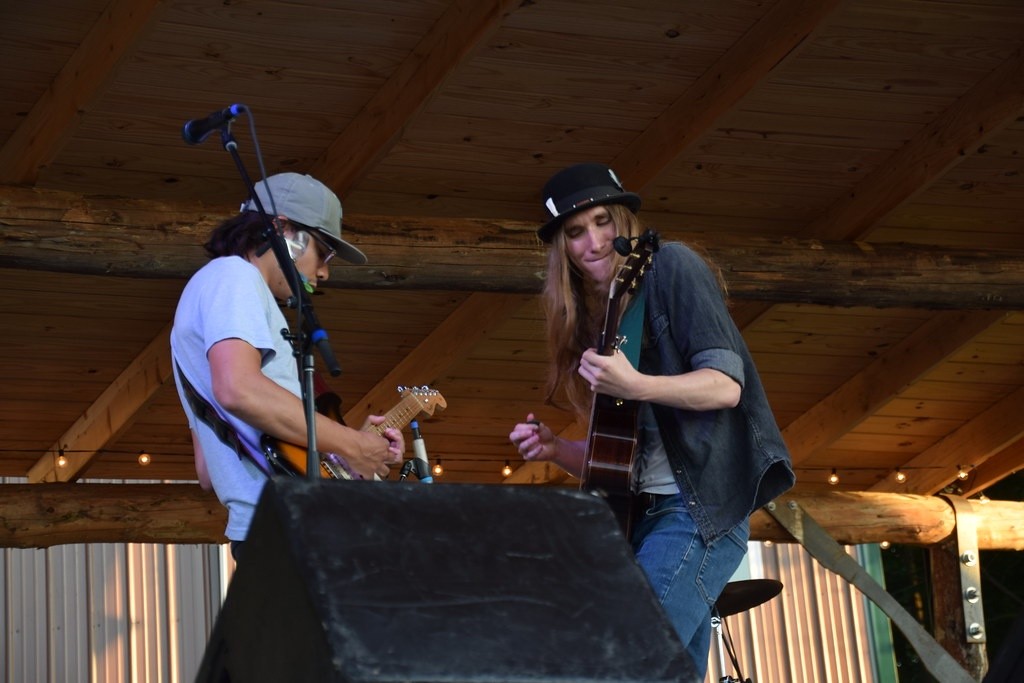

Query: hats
[240,171,368,264]
[536,161,643,245]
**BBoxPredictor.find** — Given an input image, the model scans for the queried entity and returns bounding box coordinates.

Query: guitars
[576,226,662,546]
[258,385,448,480]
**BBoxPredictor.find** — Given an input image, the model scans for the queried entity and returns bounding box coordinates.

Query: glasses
[285,220,337,265]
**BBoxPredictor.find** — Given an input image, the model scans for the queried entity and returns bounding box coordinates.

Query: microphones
[410,418,433,483]
[182,104,247,145]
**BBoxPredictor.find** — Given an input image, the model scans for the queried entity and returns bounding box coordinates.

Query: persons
[507,163,797,681]
[171,170,406,565]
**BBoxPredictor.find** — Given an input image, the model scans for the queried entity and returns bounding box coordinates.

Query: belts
[634,491,674,515]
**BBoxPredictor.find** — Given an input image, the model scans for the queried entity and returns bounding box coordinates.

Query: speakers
[196,474,702,683]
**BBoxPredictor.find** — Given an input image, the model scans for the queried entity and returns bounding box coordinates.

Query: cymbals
[709,577,784,620]
